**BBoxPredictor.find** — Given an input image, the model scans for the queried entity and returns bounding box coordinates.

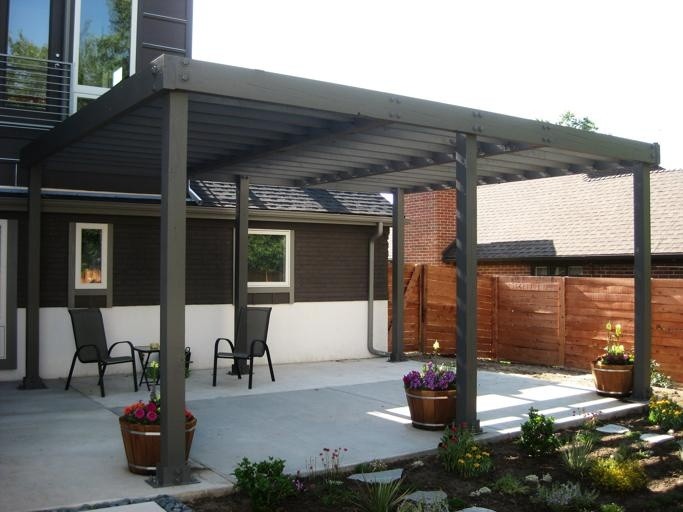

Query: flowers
[402,339,458,391]
[122,392,193,425]
[148,360,159,382]
[590,318,635,366]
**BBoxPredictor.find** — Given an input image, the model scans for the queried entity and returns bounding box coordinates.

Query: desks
[133,345,161,391]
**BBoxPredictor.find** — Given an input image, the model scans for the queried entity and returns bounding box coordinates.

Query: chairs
[212,303,276,389]
[64,305,139,398]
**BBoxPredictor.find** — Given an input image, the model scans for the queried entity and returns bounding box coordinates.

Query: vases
[118,416,198,476]
[589,361,635,398]
[405,387,457,431]
